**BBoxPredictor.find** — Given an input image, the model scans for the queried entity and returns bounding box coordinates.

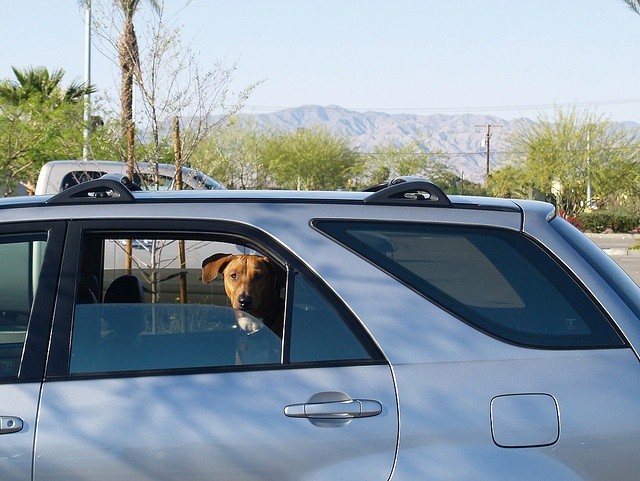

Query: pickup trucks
[1,160,264,320]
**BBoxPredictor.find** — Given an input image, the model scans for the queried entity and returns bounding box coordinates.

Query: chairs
[72,271,105,372]
[101,275,147,372]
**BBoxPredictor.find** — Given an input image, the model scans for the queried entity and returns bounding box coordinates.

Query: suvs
[0,171,640,481]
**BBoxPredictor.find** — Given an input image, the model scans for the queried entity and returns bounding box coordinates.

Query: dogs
[202,251,280,365]
